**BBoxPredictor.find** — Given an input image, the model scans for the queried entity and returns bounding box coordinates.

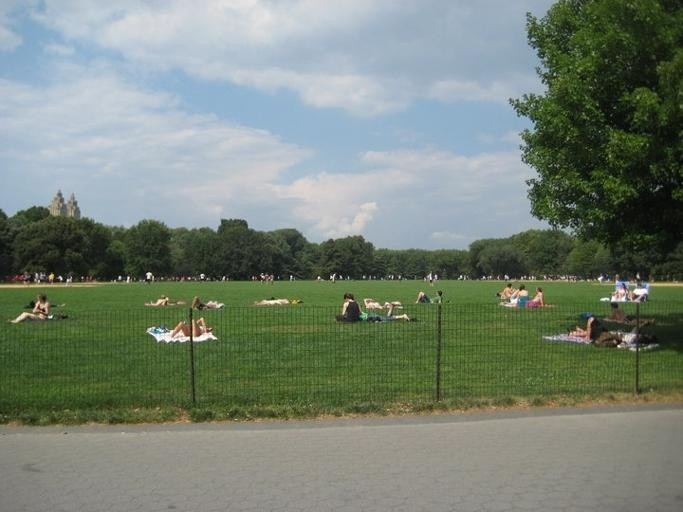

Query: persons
[11,272,226,285]
[569,273,653,344]
[11,294,52,325]
[458,273,611,283]
[335,289,444,322]
[250,272,438,287]
[144,295,303,338]
[497,281,546,308]
